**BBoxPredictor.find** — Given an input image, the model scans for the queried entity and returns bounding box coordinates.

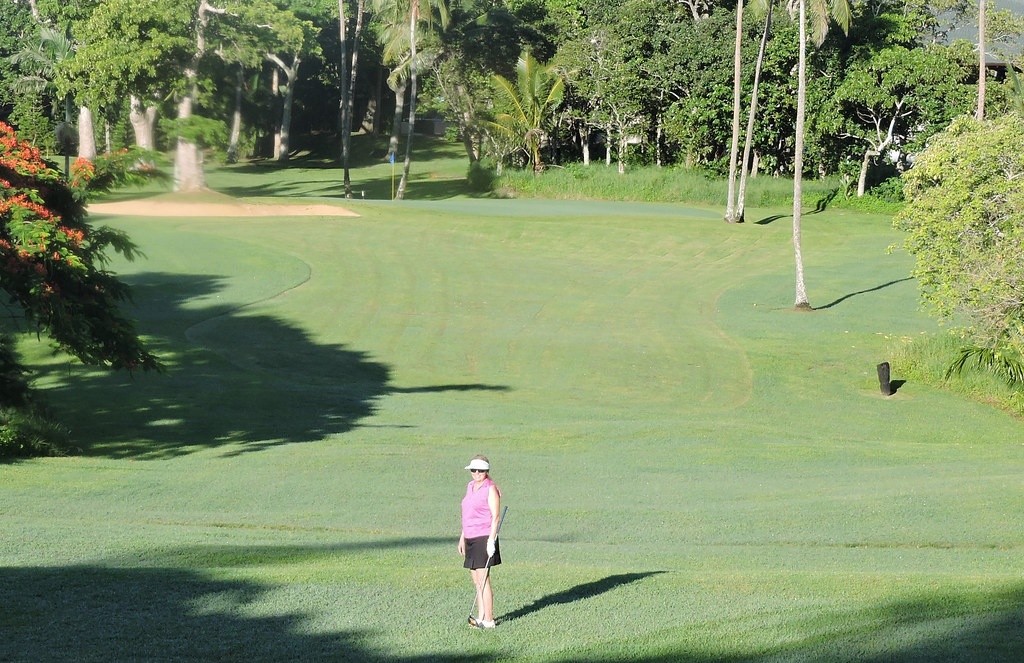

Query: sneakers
[469,619,496,629]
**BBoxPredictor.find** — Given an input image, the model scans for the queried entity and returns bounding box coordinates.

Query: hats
[464,459,490,470]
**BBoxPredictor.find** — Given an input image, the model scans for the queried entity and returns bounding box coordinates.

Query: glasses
[470,469,486,473]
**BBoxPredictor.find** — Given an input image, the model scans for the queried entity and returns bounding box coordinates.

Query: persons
[458,455,501,629]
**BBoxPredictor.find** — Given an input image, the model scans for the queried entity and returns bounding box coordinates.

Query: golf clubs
[466,504,509,628]
